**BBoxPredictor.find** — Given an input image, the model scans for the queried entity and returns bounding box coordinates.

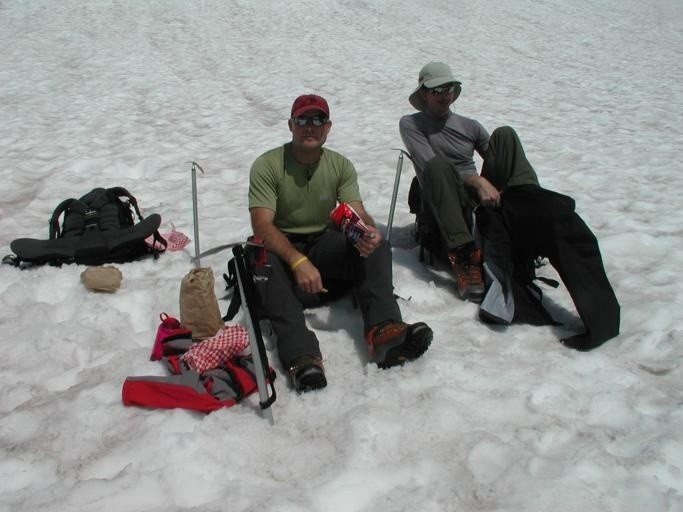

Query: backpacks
[49,186,166,265]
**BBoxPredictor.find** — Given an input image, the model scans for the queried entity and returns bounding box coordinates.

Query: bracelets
[291,257,308,269]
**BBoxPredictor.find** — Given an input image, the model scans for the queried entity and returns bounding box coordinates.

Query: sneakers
[290,355,326,394]
[447,240,488,302]
[368,321,434,369]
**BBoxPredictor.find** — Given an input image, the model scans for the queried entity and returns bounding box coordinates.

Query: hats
[291,94,330,120]
[408,61,462,110]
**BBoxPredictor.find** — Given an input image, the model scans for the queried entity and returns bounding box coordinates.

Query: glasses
[292,114,328,127]
[427,84,457,95]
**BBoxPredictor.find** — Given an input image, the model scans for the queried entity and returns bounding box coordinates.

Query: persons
[399,61,542,301]
[244,95,434,390]
